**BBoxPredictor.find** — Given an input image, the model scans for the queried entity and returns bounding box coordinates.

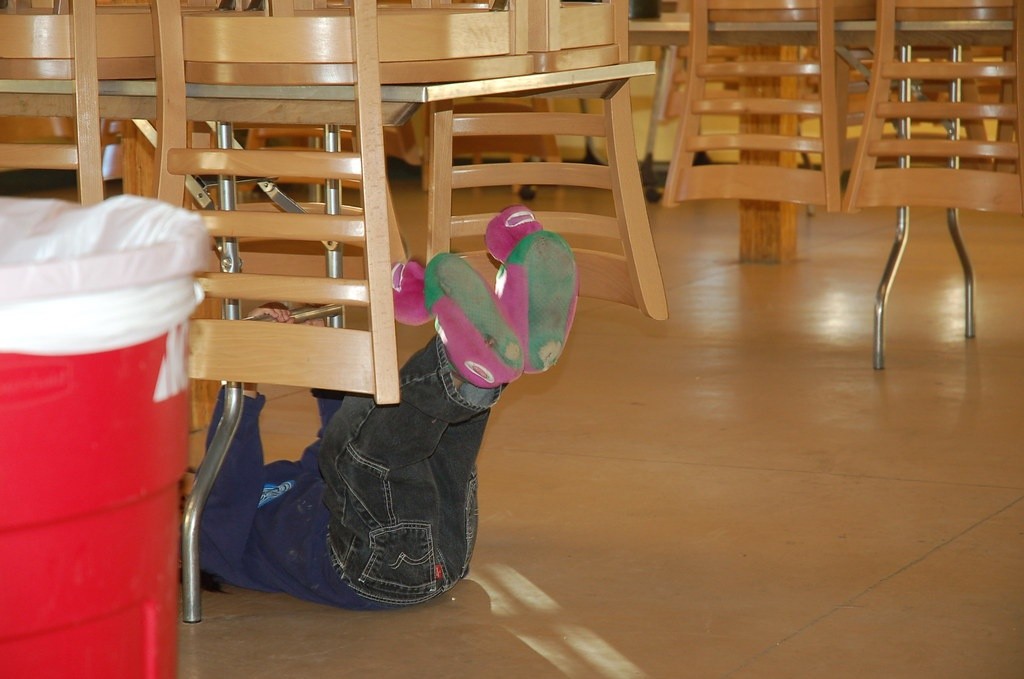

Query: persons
[177,204,580,612]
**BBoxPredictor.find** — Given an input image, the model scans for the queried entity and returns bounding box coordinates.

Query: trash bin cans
[0,194,212,679]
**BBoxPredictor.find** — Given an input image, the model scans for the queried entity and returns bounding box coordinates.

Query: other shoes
[392,252,524,389]
[484,205,580,375]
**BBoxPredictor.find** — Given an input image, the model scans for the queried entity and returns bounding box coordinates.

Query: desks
[0,61,658,622]
[624,16,1016,370]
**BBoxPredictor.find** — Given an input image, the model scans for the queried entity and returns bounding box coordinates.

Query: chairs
[0,0,1024,406]
